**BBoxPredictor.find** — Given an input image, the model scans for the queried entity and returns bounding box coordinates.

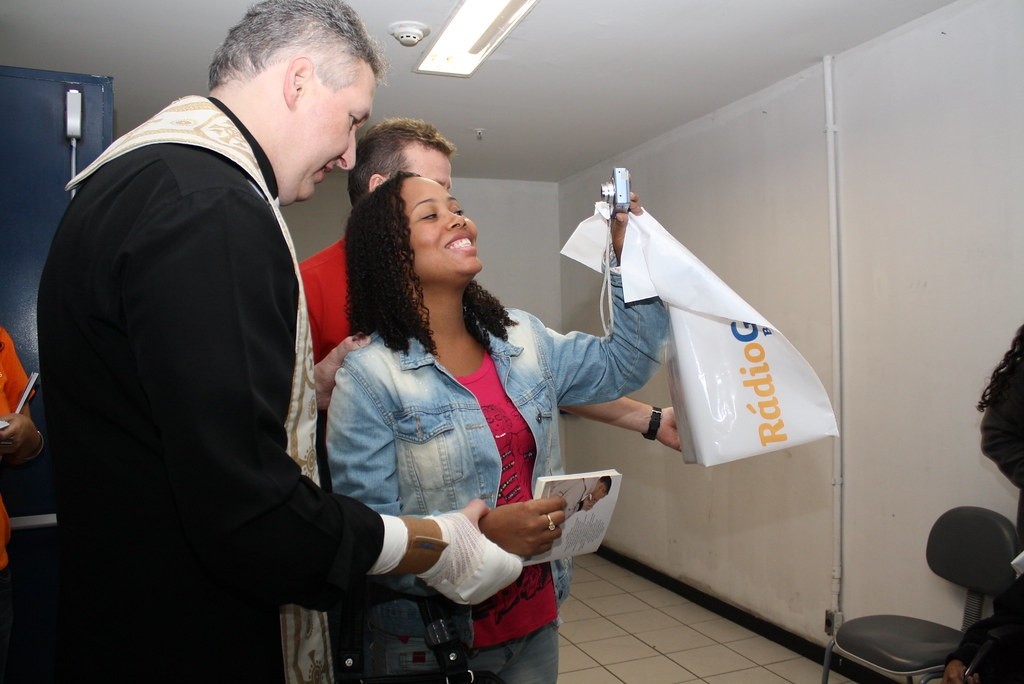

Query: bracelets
[642,407,662,441]
[24,431,44,459]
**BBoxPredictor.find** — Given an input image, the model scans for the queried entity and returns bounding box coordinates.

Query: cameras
[600,167,631,218]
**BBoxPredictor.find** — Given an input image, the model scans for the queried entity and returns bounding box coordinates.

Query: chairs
[822,507,1021,684]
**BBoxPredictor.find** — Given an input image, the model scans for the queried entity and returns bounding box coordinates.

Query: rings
[546,513,555,531]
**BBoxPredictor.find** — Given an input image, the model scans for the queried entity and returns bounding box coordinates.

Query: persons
[542,476,612,520]
[977,325,1024,548]
[36,0,522,684]
[941,572,1024,684]
[325,170,665,684]
[0,326,41,571]
[298,118,682,453]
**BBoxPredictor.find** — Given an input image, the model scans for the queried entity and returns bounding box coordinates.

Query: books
[522,468,622,566]
[0,373,39,429]
